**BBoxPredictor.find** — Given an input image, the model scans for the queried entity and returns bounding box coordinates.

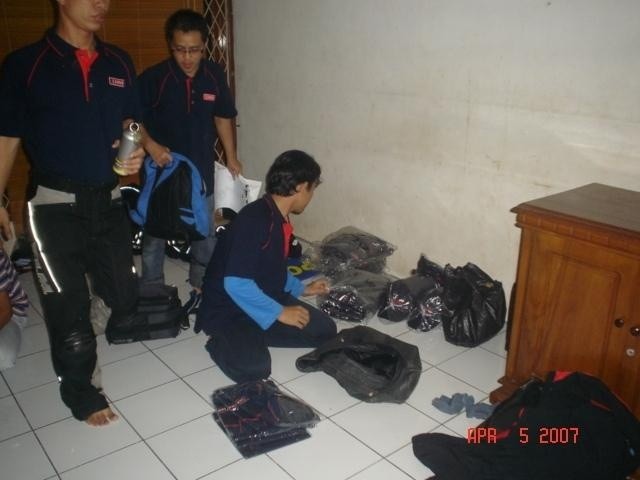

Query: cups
[113,121,142,175]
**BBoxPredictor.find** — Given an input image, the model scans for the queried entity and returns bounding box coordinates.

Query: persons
[194,150,336,384]
[136,8,241,289]
[1,1,146,426]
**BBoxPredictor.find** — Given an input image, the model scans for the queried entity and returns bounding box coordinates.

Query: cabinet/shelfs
[489,182,639,478]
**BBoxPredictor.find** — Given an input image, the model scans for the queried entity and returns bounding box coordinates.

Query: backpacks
[126,152,209,240]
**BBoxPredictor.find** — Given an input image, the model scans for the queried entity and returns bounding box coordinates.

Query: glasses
[172,45,201,52]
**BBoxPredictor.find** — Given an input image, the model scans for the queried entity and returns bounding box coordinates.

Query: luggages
[105,285,181,345]
